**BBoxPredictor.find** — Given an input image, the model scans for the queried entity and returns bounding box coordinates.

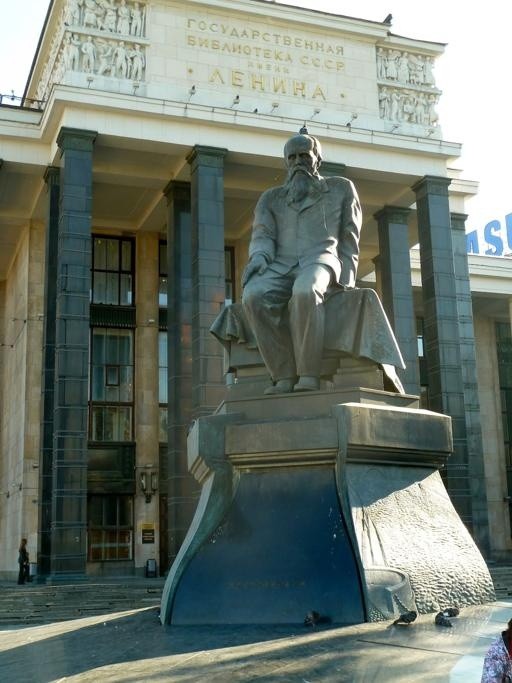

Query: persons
[480,617,512,683]
[16,538,32,585]
[242,132,364,395]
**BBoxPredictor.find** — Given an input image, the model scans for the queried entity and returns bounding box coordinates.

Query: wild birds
[391,609,417,627]
[435,606,461,630]
[304,609,320,631]
